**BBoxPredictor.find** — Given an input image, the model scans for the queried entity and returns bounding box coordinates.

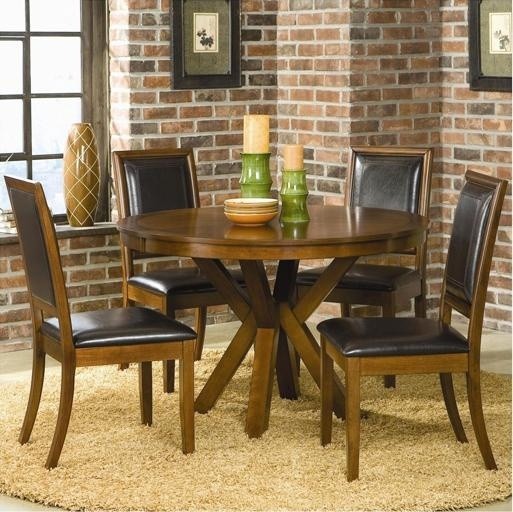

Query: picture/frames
[168,1,242,92]
[464,1,512,96]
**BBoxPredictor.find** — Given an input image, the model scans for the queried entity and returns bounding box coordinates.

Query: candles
[241,114,269,152]
[283,145,303,171]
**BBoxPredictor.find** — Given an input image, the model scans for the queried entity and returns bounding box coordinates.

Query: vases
[62,122,100,229]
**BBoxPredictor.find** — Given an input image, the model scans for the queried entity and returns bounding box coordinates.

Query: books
[0,206,57,234]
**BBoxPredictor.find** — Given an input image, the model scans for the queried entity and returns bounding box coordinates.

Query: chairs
[296,147,433,388]
[4,176,199,471]
[317,169,509,482]
[112,149,247,371]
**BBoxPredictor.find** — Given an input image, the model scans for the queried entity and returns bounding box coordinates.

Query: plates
[224,197,280,227]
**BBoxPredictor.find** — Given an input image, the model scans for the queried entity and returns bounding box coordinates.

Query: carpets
[0,344,512,506]
